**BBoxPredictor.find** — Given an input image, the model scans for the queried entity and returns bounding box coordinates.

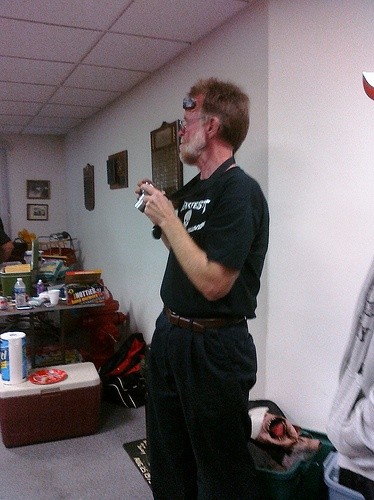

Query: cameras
[134,182,156,214]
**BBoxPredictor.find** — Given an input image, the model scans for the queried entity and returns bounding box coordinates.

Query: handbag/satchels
[98,333,146,408]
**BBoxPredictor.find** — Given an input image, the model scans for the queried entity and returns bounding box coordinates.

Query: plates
[25,251,43,255]
[28,368,66,385]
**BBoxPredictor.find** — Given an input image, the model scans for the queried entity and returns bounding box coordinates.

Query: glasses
[180,118,206,129]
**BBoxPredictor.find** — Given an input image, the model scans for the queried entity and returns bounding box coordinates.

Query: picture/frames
[26,203,49,221]
[26,180,51,199]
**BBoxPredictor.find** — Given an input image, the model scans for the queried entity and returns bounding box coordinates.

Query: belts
[164,305,245,333]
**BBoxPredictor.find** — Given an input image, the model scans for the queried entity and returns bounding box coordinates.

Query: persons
[0,220,14,263]
[135,77,269,500]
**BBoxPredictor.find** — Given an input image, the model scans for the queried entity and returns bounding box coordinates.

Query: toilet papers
[0,331,27,385]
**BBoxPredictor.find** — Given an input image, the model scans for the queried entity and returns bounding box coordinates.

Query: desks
[0,298,106,369]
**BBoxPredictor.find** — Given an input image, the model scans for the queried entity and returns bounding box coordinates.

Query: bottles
[37,280,44,296]
[13,277,26,307]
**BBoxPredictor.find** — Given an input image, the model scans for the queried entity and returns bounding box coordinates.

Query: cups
[48,289,60,306]
[0,297,8,309]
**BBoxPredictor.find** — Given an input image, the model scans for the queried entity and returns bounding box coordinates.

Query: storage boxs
[323,451,364,500]
[0,361,104,448]
[0,267,32,296]
[65,271,105,305]
[254,430,317,500]
